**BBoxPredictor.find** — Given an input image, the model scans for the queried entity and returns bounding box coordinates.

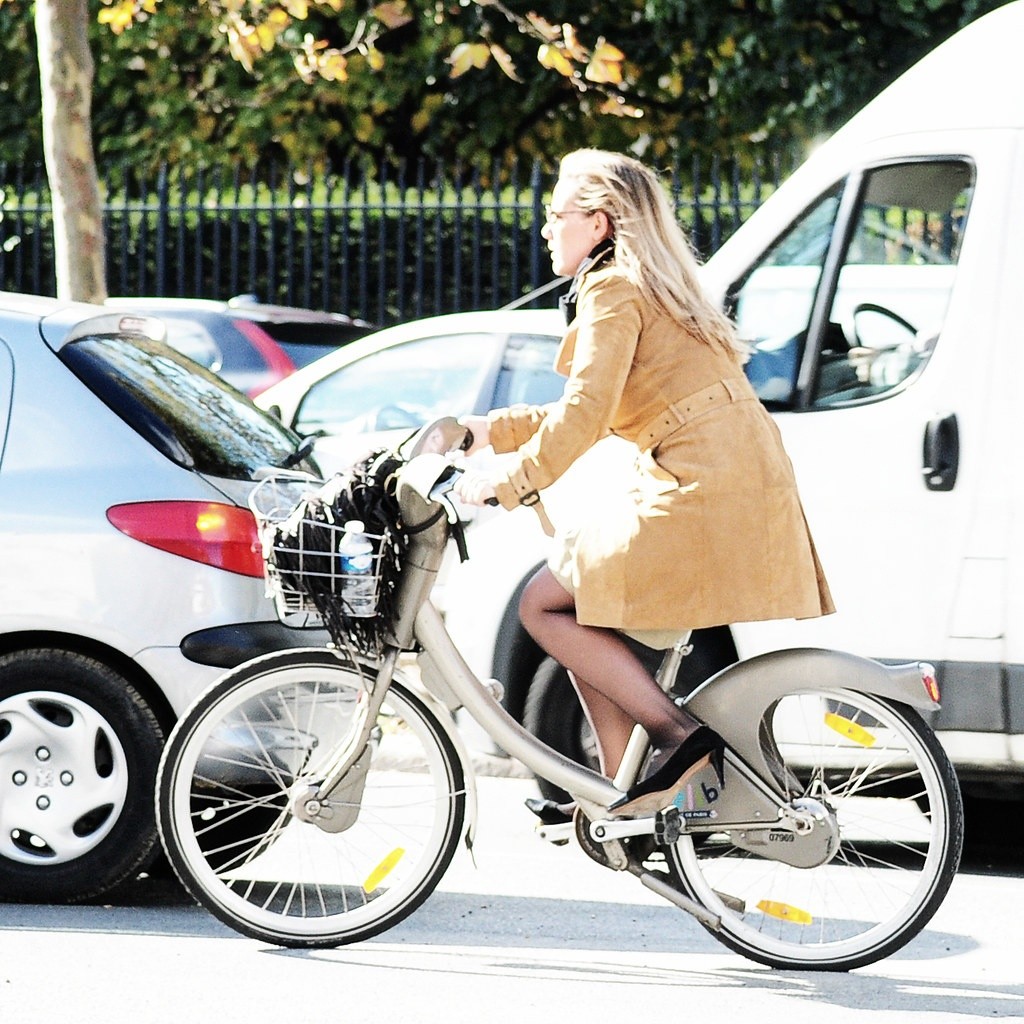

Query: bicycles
[152,416,967,969]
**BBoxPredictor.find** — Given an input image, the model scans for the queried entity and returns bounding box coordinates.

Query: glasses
[544,203,592,223]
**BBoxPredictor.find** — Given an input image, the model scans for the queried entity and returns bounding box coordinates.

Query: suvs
[0,287,336,908]
[107,292,374,409]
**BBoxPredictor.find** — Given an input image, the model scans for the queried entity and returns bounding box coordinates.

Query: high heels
[605,724,724,811]
[526,794,574,827]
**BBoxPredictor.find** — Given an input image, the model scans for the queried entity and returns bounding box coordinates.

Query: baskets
[245,474,405,630]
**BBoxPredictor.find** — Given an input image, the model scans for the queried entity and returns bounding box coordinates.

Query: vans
[409,0,1021,852]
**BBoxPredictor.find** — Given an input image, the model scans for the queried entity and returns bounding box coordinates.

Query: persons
[456,149,838,827]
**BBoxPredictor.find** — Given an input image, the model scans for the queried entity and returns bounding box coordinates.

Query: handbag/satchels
[281,445,429,647]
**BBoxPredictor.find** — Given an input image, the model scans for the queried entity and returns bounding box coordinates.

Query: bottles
[340,520,378,620]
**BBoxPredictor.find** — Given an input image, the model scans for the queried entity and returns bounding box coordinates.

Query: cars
[240,294,677,497]
